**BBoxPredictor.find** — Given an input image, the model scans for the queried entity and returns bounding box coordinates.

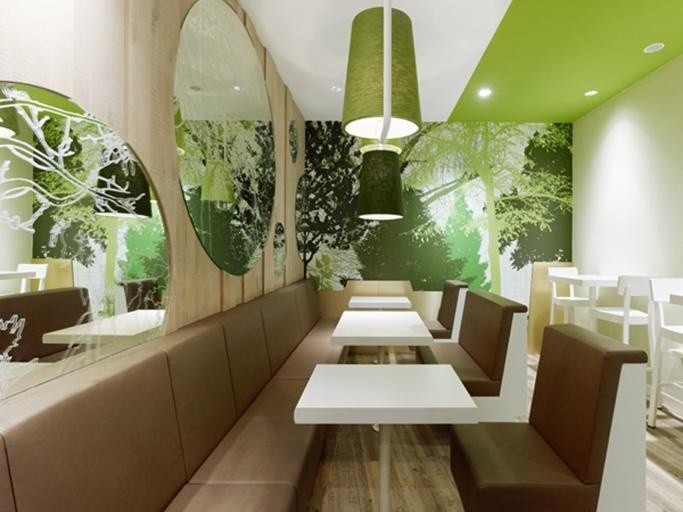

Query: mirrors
[171,1,276,277]
[0,79,171,403]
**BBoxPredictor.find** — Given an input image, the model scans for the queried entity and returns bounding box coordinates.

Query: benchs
[0,286,91,363]
[112,278,159,315]
[408,279,469,351]
[1,277,351,512]
[449,322,649,512]
[415,289,530,423]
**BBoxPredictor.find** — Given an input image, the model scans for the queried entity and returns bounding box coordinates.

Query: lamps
[341,1,422,140]
[201,158,235,204]
[354,138,407,221]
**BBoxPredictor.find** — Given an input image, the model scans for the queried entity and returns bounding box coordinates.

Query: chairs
[546,265,682,431]
[17,262,48,293]
